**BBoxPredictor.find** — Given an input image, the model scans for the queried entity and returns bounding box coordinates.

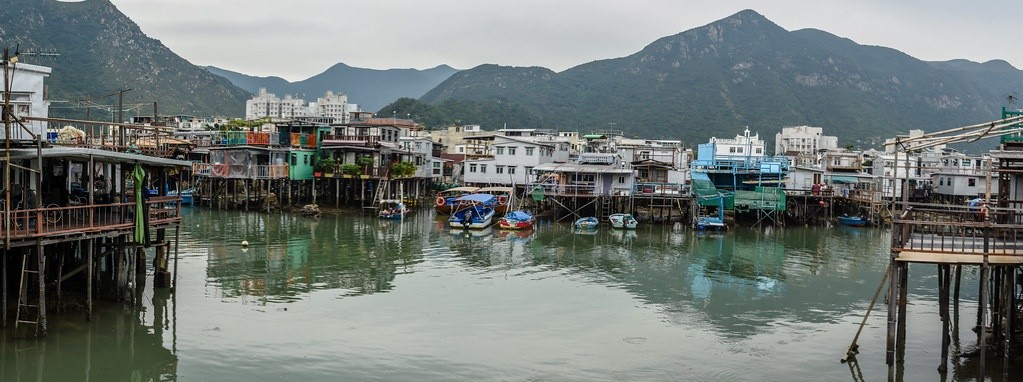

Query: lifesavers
[436,196,445,206]
[498,196,507,205]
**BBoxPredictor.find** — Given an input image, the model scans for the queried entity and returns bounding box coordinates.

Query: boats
[498,209,537,229]
[837,213,868,226]
[448,193,497,229]
[147,188,195,208]
[609,213,639,228]
[575,216,599,228]
[379,199,409,219]
